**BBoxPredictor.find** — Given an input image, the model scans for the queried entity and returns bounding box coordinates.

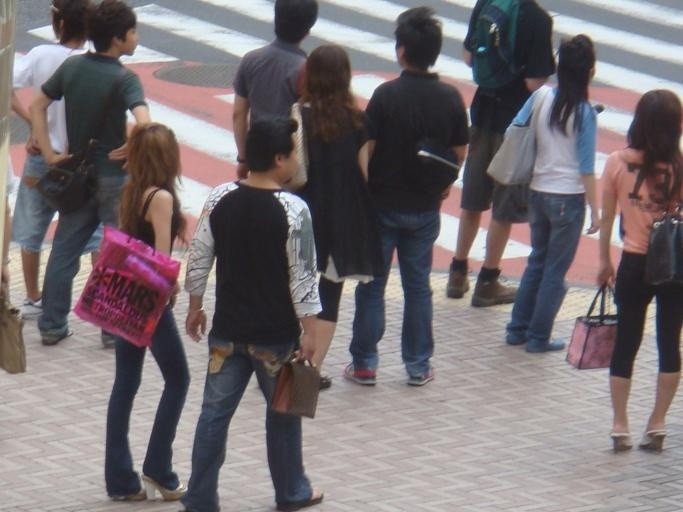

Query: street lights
[72,224,180,349]
[565,314,617,370]
[36,158,98,215]
[642,212,683,291]
[486,124,535,186]
[282,102,308,193]
[270,353,321,418]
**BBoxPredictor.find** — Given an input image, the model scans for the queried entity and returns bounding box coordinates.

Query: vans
[611,431,632,453]
[638,429,666,454]
[112,488,146,501]
[141,475,188,501]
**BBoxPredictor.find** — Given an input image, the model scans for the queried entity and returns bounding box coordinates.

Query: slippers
[468,0,519,90]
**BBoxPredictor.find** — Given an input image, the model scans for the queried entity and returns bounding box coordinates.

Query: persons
[505,35,601,353]
[29,0,151,345]
[105,123,190,503]
[10,0,103,320]
[447,0,556,306]
[287,45,369,390]
[180,115,323,512]
[343,7,469,386]
[233,0,318,179]
[596,90,683,453]
[0,146,14,296]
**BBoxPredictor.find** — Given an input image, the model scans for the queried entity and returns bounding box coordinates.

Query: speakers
[471,275,519,306]
[446,262,469,298]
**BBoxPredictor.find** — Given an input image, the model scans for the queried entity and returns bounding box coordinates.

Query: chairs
[277,486,323,512]
[344,361,376,384]
[407,366,433,386]
[16,297,44,317]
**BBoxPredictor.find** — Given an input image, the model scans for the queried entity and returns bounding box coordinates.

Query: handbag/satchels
[236,155,247,163]
[187,308,204,314]
[1,258,10,266]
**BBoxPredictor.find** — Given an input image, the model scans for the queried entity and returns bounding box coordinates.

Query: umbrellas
[291,357,331,390]
[525,337,565,353]
[506,333,526,346]
